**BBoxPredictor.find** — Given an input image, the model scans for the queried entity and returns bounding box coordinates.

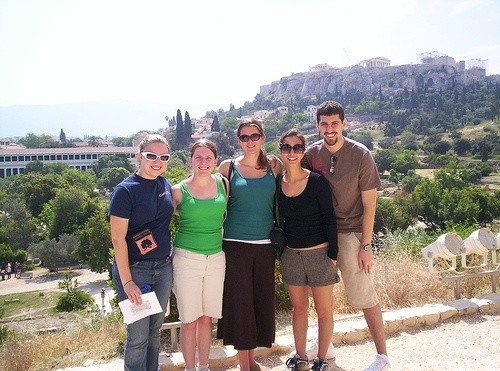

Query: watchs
[361,244,371,252]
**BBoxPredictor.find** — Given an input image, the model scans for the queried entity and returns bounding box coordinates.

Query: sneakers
[285,338,336,360]
[363,354,392,371]
[313,356,331,370]
[287,353,311,371]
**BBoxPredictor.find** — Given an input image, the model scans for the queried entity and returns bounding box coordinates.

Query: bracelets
[123,280,134,288]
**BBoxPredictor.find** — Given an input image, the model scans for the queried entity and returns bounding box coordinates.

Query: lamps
[420,228,500,274]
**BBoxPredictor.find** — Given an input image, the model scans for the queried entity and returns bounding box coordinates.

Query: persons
[169,138,231,371]
[217,119,282,370]
[286,101,391,371]
[276,128,342,371]
[109,134,174,371]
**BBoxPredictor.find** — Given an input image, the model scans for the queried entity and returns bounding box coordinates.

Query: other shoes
[251,361,263,371]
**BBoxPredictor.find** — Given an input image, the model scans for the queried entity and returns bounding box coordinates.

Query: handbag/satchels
[132,228,160,256]
[270,174,287,248]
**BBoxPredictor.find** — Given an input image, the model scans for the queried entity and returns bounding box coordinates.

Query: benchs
[17,271,33,279]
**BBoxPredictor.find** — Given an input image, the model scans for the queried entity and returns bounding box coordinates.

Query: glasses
[140,151,170,163]
[329,153,338,176]
[238,133,262,143]
[279,144,306,154]
